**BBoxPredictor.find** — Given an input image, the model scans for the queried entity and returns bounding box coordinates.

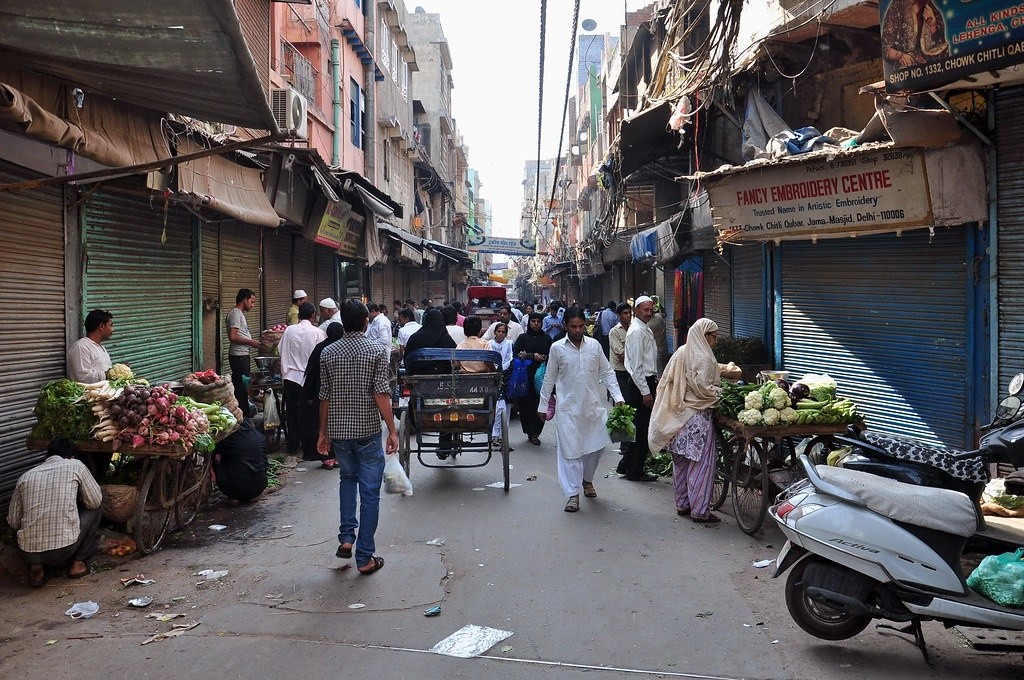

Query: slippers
[28,573,43,587]
[678,506,691,515]
[335,545,352,559]
[693,513,721,522]
[360,555,384,575]
[68,561,90,578]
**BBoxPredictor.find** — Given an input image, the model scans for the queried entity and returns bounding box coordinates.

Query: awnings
[0,0,475,272]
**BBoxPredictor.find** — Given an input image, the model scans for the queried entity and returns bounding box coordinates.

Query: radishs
[74,380,125,442]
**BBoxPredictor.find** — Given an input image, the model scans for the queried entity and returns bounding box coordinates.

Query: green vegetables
[807,385,836,402]
[267,454,284,486]
[30,379,94,441]
[645,449,674,477]
[606,405,637,441]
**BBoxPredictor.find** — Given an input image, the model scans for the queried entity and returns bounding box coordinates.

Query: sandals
[563,495,579,512]
[582,479,597,497]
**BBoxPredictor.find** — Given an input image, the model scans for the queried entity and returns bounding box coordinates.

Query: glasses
[709,332,716,339]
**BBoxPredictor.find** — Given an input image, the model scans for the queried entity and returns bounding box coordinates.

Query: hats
[320,297,336,308]
[635,295,653,308]
[294,289,307,298]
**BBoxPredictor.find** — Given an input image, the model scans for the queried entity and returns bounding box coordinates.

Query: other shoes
[321,463,339,470]
[285,448,297,454]
[492,439,502,446]
[616,463,658,481]
[435,448,447,460]
[527,433,541,444]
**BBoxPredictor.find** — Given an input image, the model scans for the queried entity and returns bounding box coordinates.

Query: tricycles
[397,346,515,494]
[467,285,507,324]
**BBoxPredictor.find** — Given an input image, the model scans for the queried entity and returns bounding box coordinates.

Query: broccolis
[737,380,800,426]
[107,363,134,379]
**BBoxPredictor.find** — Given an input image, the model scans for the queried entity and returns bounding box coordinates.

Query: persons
[7,438,103,584]
[537,308,626,513]
[68,308,129,384]
[225,289,261,418]
[664,317,724,523]
[209,391,269,505]
[317,300,398,575]
[280,289,667,481]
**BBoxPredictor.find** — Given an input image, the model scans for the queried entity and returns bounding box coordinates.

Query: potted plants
[736,337,772,382]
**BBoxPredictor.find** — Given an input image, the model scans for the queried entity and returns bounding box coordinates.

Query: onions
[271,323,288,330]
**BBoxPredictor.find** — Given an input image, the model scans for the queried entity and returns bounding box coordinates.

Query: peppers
[720,382,762,420]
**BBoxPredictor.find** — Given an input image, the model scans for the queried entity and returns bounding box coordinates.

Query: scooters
[394,357,411,420]
[747,435,1024,665]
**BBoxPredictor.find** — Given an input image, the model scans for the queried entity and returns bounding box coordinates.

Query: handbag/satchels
[592,312,603,340]
[508,358,532,397]
[262,388,281,431]
[534,360,556,421]
[383,453,413,497]
[609,419,637,444]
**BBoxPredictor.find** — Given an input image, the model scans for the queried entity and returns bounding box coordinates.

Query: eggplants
[773,378,817,409]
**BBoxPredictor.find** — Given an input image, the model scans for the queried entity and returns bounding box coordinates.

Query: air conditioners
[268,88,309,139]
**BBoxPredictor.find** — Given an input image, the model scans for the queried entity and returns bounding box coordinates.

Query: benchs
[403,373,504,423]
